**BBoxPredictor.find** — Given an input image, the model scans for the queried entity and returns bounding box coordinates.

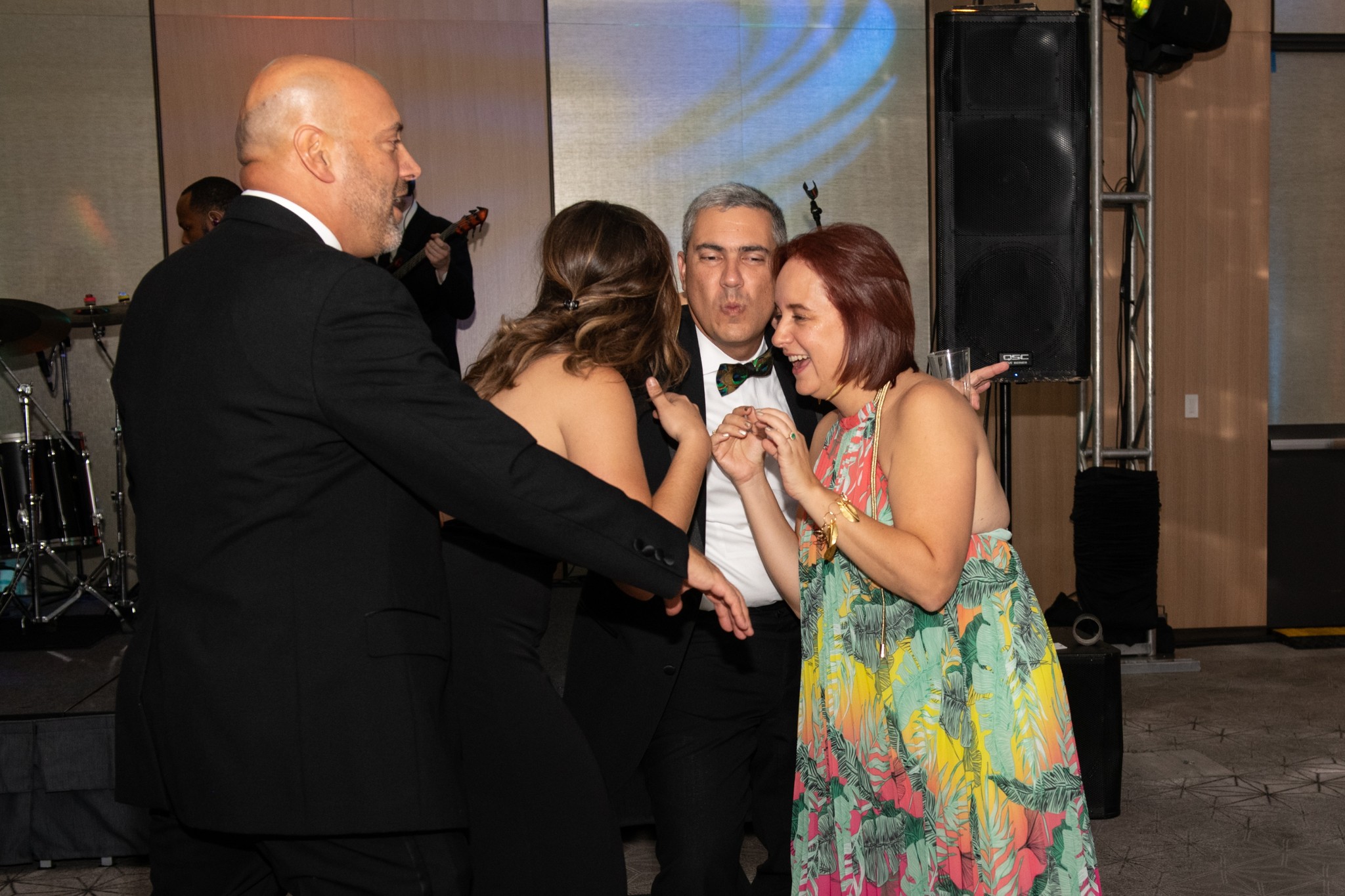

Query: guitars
[390,205,489,281]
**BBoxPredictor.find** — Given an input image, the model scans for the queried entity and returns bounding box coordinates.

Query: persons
[368,175,477,379]
[439,199,713,895]
[616,181,1011,896]
[110,53,754,896]
[705,223,1104,896]
[175,175,244,247]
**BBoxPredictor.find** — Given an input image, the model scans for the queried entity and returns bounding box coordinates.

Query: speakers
[922,4,1105,394]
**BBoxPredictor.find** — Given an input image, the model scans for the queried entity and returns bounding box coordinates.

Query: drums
[0,427,105,567]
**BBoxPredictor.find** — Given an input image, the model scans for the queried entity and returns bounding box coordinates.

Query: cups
[927,347,970,404]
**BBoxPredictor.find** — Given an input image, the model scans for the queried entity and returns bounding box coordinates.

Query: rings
[786,432,796,440]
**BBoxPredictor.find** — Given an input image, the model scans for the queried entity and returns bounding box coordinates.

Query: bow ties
[716,348,774,397]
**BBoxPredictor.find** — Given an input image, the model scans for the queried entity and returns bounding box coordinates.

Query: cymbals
[58,303,130,330]
[0,297,73,365]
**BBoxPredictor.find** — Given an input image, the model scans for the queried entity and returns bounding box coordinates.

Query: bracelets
[812,494,860,564]
